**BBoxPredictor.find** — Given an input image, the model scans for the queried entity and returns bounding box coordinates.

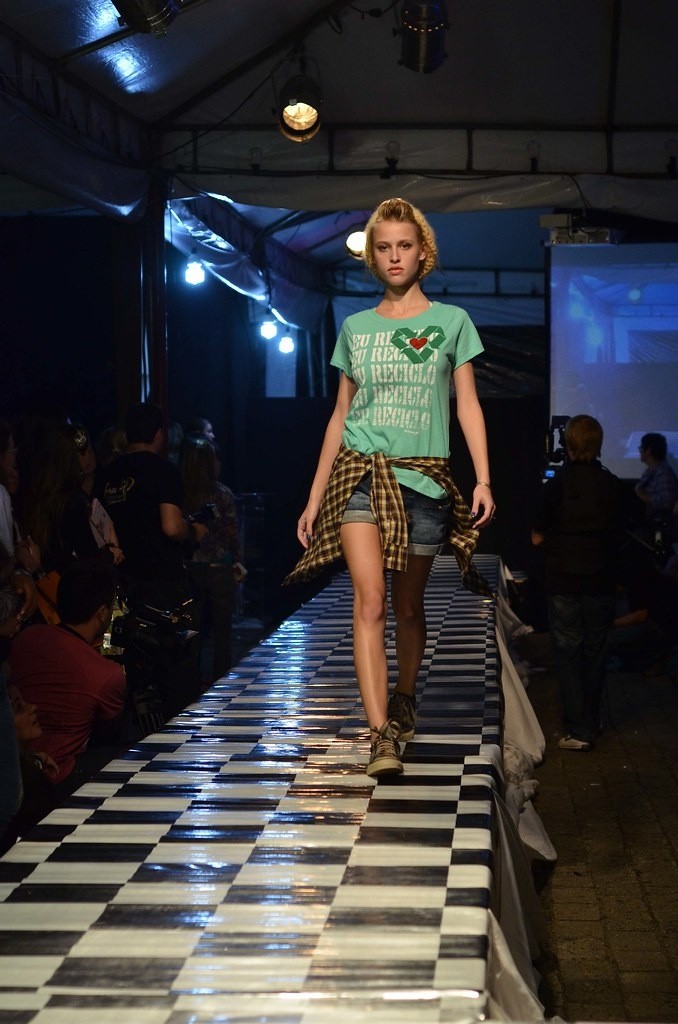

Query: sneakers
[366,721,404,776]
[386,688,416,741]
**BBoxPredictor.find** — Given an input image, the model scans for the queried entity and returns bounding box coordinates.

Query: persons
[298,198,496,778]
[531,415,678,749]
[0,402,248,833]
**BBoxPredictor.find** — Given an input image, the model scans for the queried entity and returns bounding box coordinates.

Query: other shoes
[560,733,589,749]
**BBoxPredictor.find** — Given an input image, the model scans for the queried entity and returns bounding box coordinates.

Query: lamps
[112,0,178,33]
[269,76,323,143]
[399,0,448,74]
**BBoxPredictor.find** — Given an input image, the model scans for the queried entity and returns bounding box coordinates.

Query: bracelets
[476,482,490,487]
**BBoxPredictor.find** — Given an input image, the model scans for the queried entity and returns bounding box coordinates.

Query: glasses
[638,447,643,450]
[4,447,18,455]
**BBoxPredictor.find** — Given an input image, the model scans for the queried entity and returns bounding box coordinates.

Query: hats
[365,198,437,282]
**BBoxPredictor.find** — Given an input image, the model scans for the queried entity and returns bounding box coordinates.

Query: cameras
[180,504,221,560]
